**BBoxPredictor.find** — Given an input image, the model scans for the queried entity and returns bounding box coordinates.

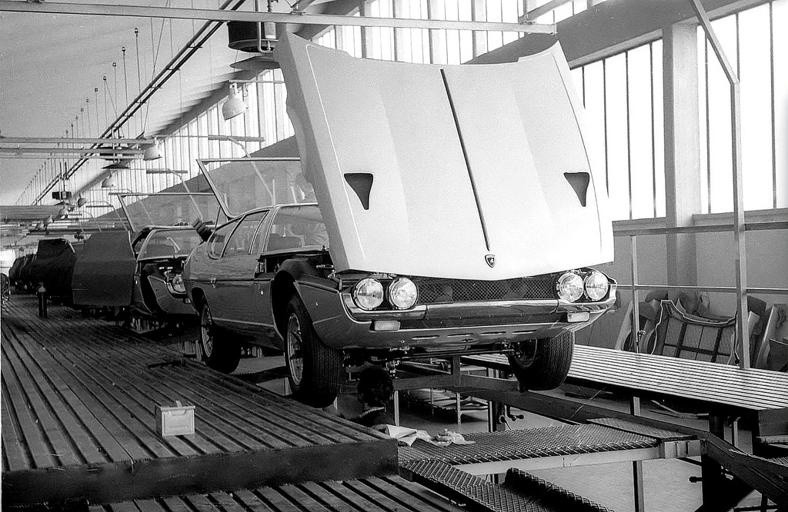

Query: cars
[182,29,618,405]
[67,190,228,320]
[9,236,131,312]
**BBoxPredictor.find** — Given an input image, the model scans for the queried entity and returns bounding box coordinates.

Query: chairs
[279,236,301,250]
[245,232,280,255]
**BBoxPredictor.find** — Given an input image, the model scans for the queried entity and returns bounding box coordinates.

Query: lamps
[78,188,154,231]
[102,167,205,224]
[36,191,69,231]
[144,135,276,207]
[222,78,286,122]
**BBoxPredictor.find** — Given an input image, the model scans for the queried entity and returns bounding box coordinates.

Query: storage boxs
[155,401,197,436]
